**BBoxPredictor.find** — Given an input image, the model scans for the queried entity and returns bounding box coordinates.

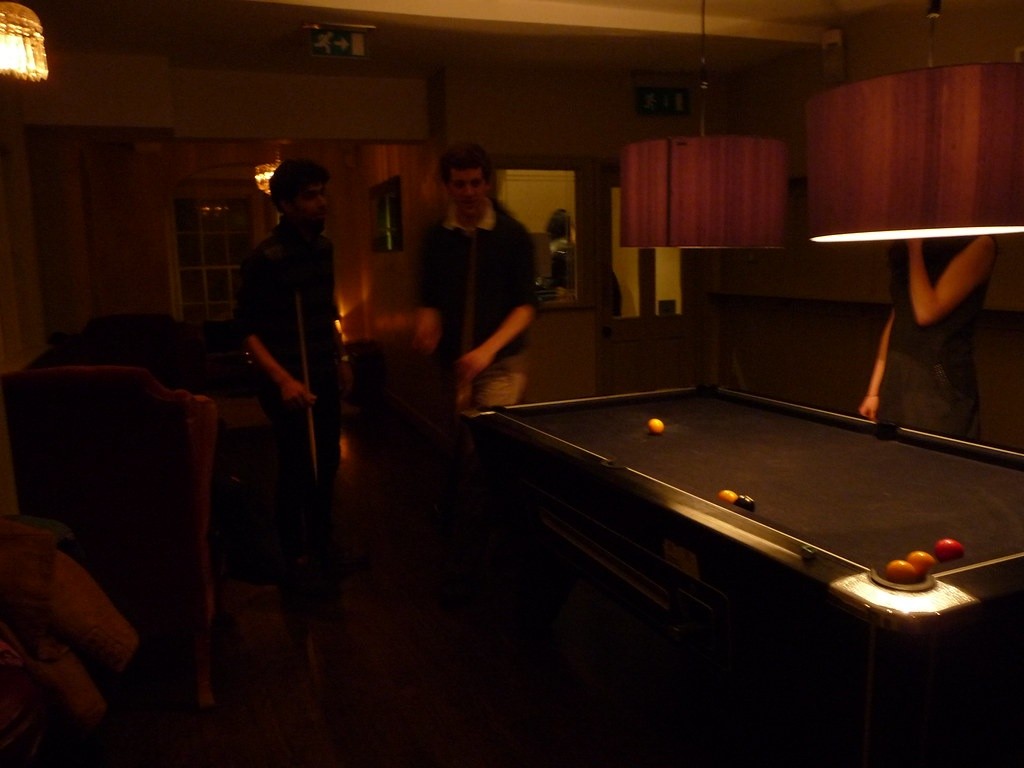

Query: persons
[408,143,530,434]
[229,157,362,610]
[535,207,622,317]
[857,231,999,442]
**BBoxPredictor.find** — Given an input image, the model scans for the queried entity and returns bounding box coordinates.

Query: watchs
[339,354,356,365]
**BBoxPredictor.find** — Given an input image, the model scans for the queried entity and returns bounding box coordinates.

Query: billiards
[718,489,757,513]
[935,538,965,563]
[649,418,665,435]
[884,550,938,585]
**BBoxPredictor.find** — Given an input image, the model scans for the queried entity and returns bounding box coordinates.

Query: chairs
[4,365,224,710]
[47,313,244,391]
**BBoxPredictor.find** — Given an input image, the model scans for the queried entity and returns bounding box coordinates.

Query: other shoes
[436,573,475,609]
[326,550,369,570]
[294,593,356,613]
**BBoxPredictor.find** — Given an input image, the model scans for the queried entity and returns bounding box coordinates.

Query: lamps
[804,0,1024,243]
[1,1,52,84]
[619,0,791,253]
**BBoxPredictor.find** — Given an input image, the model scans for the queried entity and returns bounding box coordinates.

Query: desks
[460,382,1024,768]
[207,386,297,578]
